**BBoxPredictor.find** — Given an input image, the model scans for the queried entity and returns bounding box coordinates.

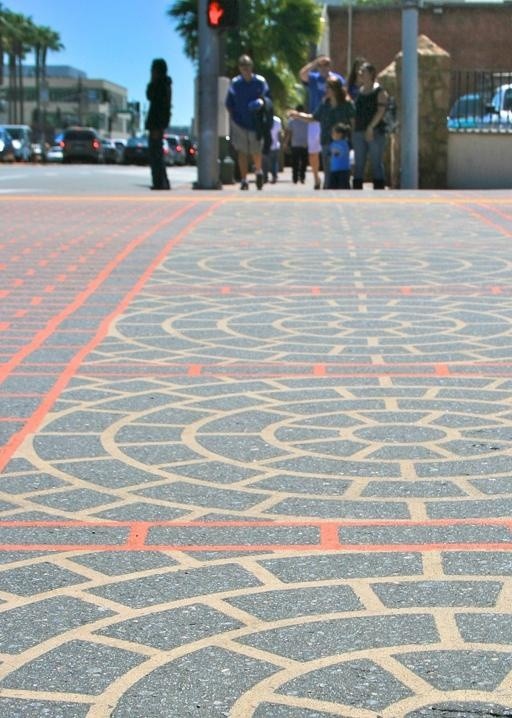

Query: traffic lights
[205,0,240,29]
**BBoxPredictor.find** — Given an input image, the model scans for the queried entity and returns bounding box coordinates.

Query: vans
[446,82,512,130]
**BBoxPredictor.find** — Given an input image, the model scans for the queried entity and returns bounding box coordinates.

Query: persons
[262,115,282,184]
[286,55,389,189]
[146,59,171,189]
[225,53,273,189]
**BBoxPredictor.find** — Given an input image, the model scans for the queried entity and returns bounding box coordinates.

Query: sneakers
[150,180,170,190]
[240,174,278,191]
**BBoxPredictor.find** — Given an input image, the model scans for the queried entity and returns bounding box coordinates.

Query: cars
[0,122,197,165]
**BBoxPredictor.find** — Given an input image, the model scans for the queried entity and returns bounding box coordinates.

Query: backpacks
[373,86,399,133]
